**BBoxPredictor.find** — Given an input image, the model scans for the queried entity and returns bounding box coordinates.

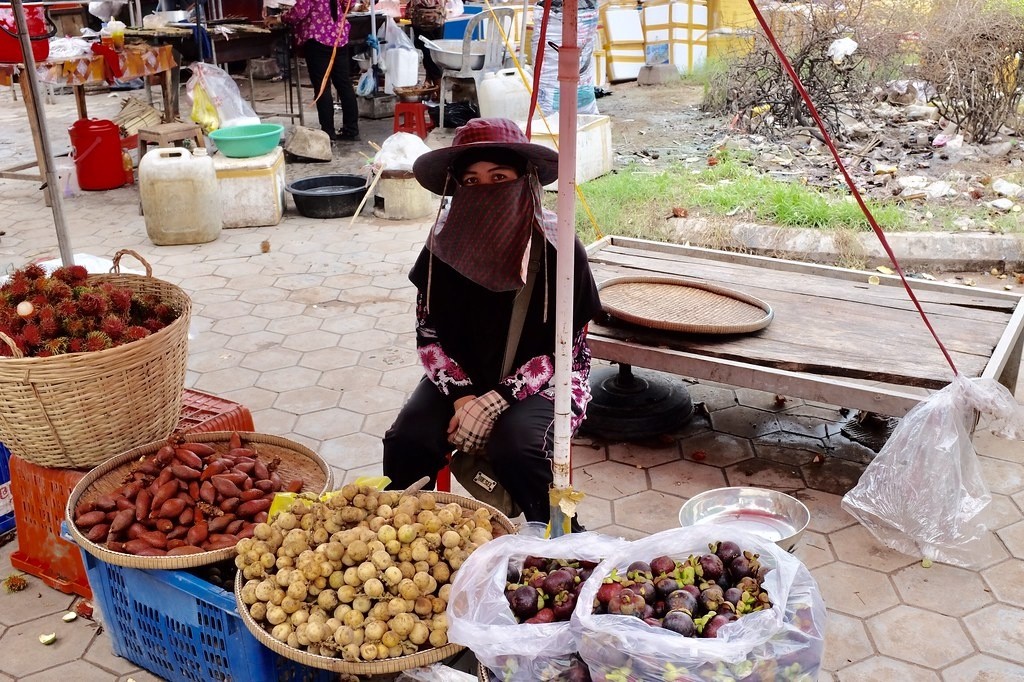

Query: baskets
[0,248,193,468]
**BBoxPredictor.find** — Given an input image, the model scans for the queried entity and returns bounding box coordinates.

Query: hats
[412,118,558,196]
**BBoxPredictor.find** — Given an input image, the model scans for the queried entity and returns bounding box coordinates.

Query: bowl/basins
[152,9,192,23]
[423,39,505,70]
[394,86,437,102]
[679,486,811,553]
[351,53,370,70]
[208,122,285,159]
[285,173,371,219]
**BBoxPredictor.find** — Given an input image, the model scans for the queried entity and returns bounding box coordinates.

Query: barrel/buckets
[67,119,125,191]
[0,2,57,62]
[137,146,222,246]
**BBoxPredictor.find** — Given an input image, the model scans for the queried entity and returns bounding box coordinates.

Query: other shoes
[334,133,361,142]
[329,133,336,141]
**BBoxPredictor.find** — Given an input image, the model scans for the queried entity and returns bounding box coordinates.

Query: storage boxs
[211,144,288,229]
[0,388,362,682]
[444,0,823,91]
[517,111,614,191]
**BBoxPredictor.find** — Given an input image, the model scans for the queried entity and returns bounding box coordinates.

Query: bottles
[101,23,114,51]
[122,147,134,185]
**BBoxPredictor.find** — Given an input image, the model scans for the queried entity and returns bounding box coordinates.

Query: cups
[108,25,124,51]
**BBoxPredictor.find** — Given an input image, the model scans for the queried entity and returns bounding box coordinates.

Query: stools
[137,122,206,217]
[393,101,435,140]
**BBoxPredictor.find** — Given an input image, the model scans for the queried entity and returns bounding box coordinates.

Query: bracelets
[277,14,282,24]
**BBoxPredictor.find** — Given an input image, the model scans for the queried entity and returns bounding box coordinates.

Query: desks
[0,44,185,206]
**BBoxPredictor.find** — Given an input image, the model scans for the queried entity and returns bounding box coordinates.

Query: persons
[381,118,605,533]
[264,0,362,140]
[403,0,446,103]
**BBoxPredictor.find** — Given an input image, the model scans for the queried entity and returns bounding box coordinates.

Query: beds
[581,235,1024,446]
